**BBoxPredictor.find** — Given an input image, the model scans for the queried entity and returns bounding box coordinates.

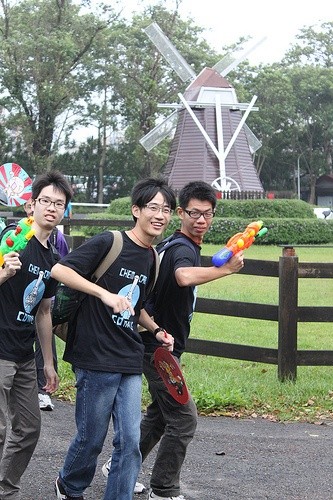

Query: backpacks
[51,229,160,342]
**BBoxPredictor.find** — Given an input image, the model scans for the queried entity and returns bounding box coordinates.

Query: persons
[0,170,75,500]
[51,177,174,500]
[102,181,244,500]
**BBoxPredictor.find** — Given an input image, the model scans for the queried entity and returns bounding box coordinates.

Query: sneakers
[149,488,186,500]
[54,475,84,500]
[38,393,54,411]
[101,457,146,493]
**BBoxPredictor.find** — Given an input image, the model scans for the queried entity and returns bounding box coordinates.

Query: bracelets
[153,328,166,340]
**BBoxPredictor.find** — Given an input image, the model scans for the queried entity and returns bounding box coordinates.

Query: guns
[214,216,269,267]
[0,215,36,267]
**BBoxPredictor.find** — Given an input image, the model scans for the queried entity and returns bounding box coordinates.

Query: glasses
[183,209,216,219]
[143,204,174,216]
[34,197,68,210]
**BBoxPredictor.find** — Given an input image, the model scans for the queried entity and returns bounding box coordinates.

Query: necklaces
[132,230,152,251]
